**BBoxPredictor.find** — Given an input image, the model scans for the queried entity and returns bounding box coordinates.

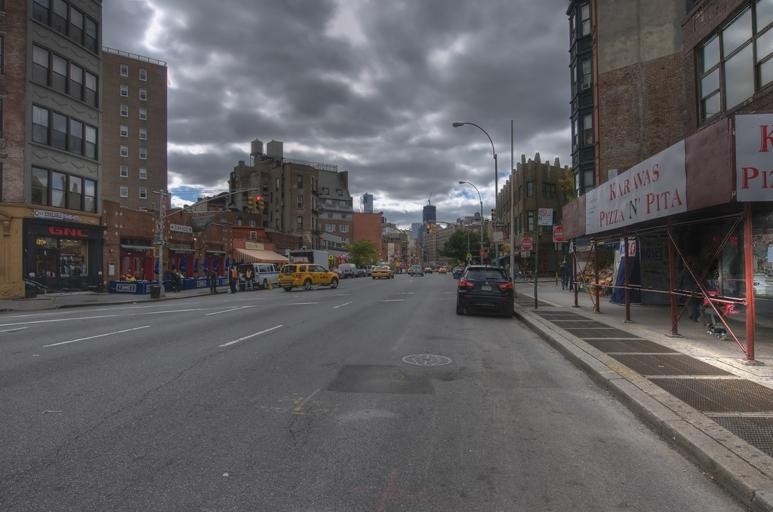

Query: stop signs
[520,237,532,250]
[552,225,568,243]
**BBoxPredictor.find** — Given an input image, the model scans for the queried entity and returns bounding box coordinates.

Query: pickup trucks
[410,265,423,277]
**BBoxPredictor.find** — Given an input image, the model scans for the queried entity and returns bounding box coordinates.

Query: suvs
[455,265,513,319]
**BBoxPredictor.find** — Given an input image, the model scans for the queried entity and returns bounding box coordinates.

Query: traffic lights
[255,196,260,209]
[247,196,252,210]
[260,197,264,209]
[426,224,429,234]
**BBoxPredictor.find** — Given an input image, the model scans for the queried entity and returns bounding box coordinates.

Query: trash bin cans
[150,285,161,298]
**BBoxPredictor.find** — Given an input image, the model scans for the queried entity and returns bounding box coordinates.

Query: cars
[438,267,446,273]
[407,267,412,274]
[453,267,464,279]
[424,267,432,274]
[371,266,393,279]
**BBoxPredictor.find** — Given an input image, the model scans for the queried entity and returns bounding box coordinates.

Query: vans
[237,263,280,288]
[338,263,373,278]
[278,263,339,291]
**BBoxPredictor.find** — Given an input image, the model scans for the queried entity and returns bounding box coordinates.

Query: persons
[682,254,712,320]
[211,266,255,295]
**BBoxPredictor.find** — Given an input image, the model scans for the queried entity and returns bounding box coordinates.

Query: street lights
[458,180,483,265]
[452,121,498,266]
